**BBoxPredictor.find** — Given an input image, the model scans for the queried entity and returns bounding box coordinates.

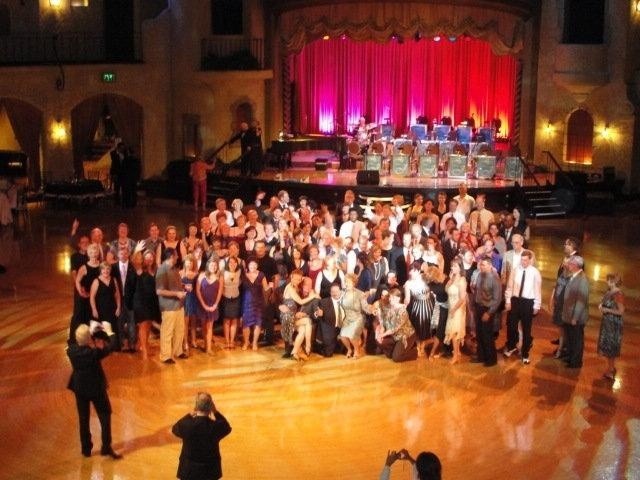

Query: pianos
[270,133,344,169]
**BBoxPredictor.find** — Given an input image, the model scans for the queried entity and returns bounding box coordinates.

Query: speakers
[356,169,380,186]
[0,150,28,177]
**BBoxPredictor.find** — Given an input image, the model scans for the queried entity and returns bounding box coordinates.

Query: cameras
[395,453,402,461]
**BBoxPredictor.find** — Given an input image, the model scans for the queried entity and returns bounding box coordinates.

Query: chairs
[11,190,31,224]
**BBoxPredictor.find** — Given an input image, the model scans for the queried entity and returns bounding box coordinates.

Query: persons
[110,143,141,209]
[67,183,544,366]
[378,449,443,480]
[59,320,123,461]
[170,391,231,479]
[551,237,626,390]
[352,116,373,154]
[229,124,258,199]
[189,150,217,212]
